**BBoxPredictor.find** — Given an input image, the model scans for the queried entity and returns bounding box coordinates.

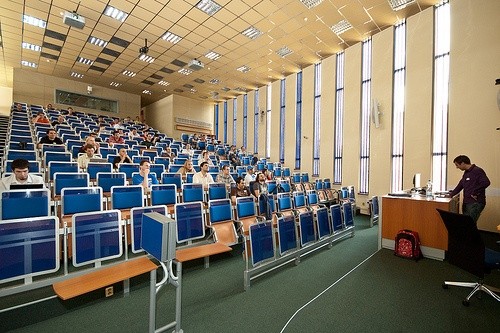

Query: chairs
[436,208,500,306]
[0,102,377,298]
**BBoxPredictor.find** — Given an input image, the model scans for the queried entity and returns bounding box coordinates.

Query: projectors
[188,59,205,71]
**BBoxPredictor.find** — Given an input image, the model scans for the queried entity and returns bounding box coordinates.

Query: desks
[381,189,460,263]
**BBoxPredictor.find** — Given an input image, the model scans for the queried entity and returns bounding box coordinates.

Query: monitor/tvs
[414,173,421,191]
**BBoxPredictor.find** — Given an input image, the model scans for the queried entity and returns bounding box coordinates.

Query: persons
[253,173,272,220]
[229,154,241,170]
[182,143,194,157]
[96,114,160,148]
[175,160,196,177]
[78,143,101,169]
[193,162,214,191]
[47,103,55,110]
[244,165,257,187]
[113,148,133,169]
[228,144,236,154]
[161,146,175,162]
[192,133,225,153]
[52,115,67,126]
[258,165,273,180]
[63,107,77,116]
[231,177,257,205]
[216,165,236,190]
[238,146,247,155]
[32,111,49,123]
[0,159,46,198]
[12,103,27,113]
[444,155,491,222]
[77,136,100,154]
[132,159,158,188]
[37,129,64,149]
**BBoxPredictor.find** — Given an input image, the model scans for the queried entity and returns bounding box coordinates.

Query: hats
[247,165,253,170]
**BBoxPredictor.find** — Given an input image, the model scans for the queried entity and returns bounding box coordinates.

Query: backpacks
[394,230,422,260]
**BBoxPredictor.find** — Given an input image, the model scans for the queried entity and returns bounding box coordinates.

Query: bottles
[426,180,433,200]
